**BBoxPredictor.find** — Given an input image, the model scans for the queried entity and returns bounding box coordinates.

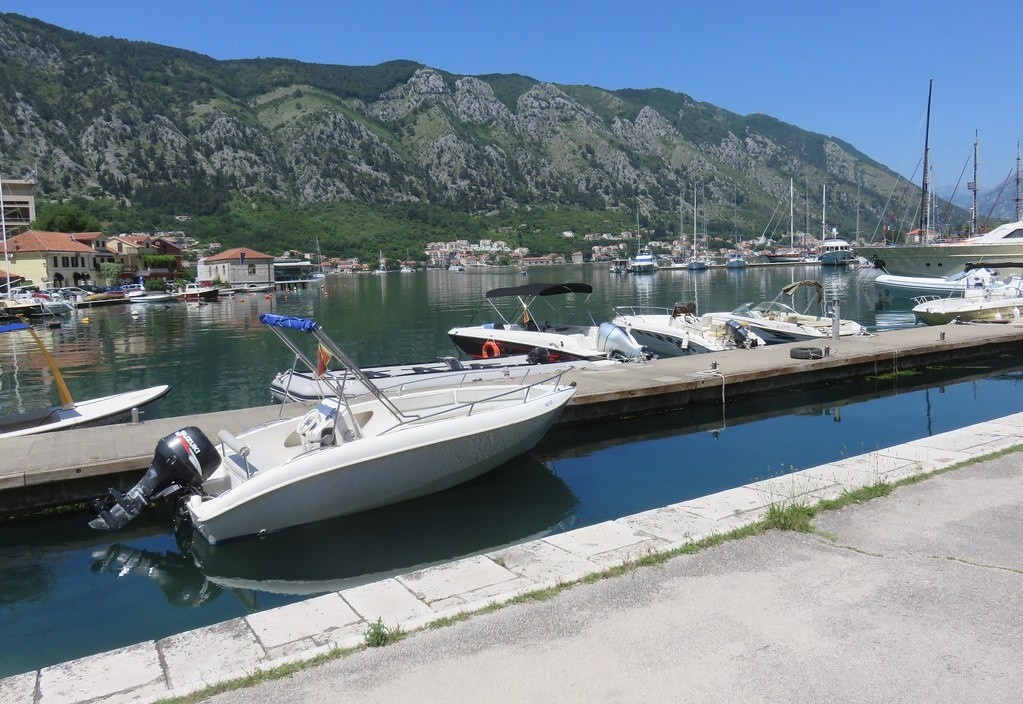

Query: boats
[88,374,577,544]
[265,348,597,407]
[700,279,870,345]
[446,283,662,363]
[609,302,768,357]
[0,169,326,332]
[80,450,585,607]
[369,77,1023,360]
[909,262,1023,331]
[0,316,172,439]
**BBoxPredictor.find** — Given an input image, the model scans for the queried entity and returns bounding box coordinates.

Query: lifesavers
[790,347,822,359]
[482,341,500,358]
